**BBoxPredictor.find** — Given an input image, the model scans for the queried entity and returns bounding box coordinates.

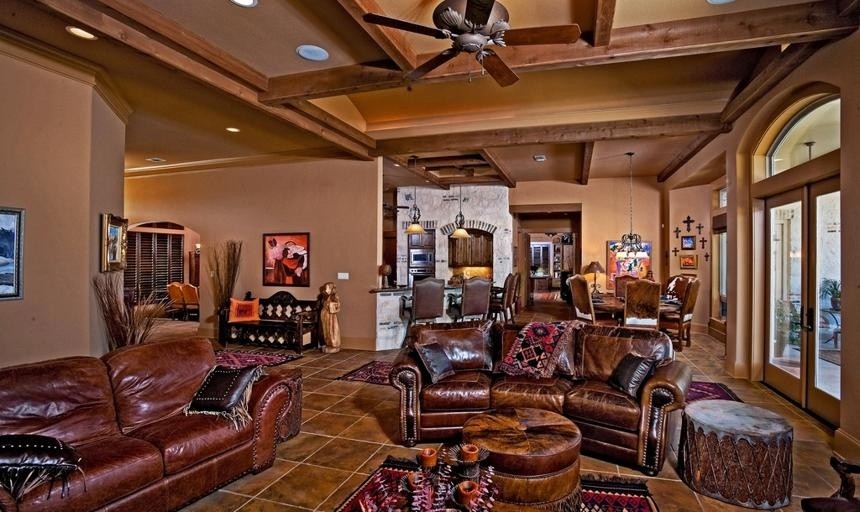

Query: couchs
[1,336,297,511]
[217,289,324,355]
[389,320,693,477]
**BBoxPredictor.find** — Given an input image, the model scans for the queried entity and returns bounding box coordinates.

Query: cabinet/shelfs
[552,243,574,287]
[533,277,552,293]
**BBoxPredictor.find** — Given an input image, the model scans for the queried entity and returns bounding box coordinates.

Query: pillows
[552,348,576,379]
[414,343,455,385]
[190,365,260,412]
[608,352,658,400]
[228,295,262,323]
[0,435,79,473]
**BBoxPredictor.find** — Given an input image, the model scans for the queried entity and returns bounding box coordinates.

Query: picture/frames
[681,236,696,250]
[606,240,652,289]
[262,231,310,287]
[0,205,27,316]
[100,212,128,273]
[680,255,699,269]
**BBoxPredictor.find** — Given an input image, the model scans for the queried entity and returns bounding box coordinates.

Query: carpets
[686,381,744,405]
[334,452,660,512]
[334,360,394,385]
[215,349,307,367]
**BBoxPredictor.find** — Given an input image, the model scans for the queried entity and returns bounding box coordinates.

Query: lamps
[404,203,427,234]
[618,233,643,257]
[450,211,471,239]
[195,244,200,254]
[584,261,605,298]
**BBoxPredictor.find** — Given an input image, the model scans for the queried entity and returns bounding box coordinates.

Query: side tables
[677,400,794,509]
[260,366,303,444]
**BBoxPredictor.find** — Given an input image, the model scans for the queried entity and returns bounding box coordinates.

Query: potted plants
[819,277,841,311]
[200,239,243,343]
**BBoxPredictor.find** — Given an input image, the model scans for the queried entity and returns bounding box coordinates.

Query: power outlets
[337,273,350,280]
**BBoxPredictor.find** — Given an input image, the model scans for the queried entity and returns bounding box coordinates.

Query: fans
[362,0,581,88]
[383,203,410,213]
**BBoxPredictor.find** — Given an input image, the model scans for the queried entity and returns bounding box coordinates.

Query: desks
[821,308,842,344]
[593,296,680,314]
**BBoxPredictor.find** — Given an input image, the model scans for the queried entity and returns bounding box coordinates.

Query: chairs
[446,276,491,324]
[569,274,619,326]
[399,277,446,348]
[182,284,200,321]
[615,274,639,297]
[490,273,513,324]
[624,280,661,331]
[168,284,187,321]
[665,275,689,302]
[497,273,520,324]
[660,277,701,352]
